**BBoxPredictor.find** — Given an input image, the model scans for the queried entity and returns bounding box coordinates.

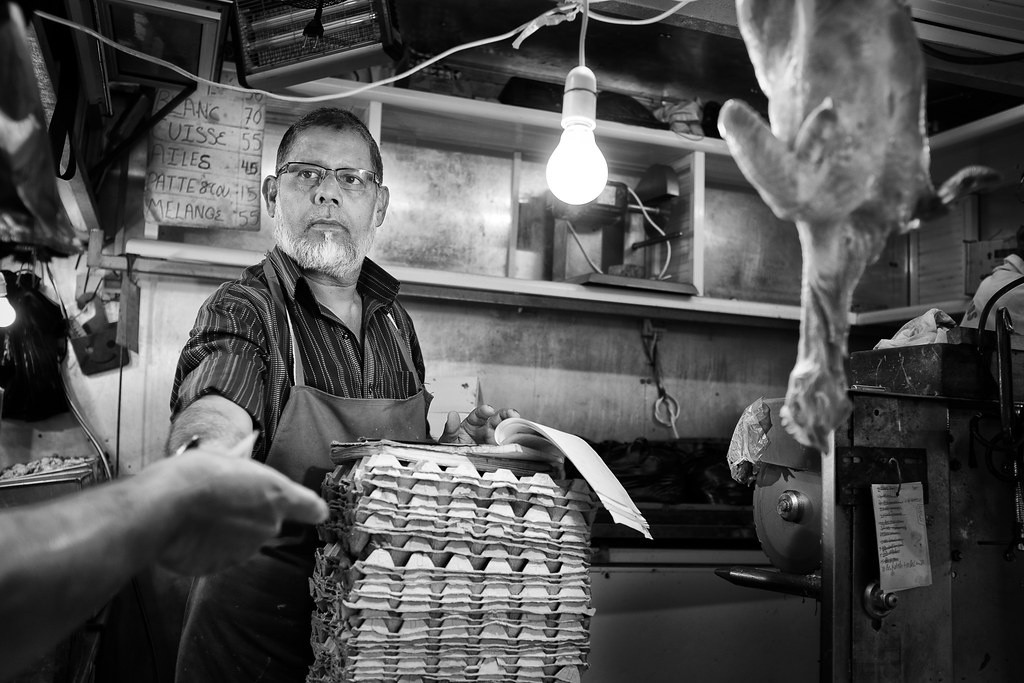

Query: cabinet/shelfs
[87,55,1024,329]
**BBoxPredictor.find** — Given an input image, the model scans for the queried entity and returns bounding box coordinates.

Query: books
[331,418,653,540]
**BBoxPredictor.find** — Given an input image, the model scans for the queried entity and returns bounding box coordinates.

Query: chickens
[717,0,993,457]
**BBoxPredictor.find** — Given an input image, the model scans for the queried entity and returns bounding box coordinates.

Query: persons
[0,450,329,683]
[164,106,522,683]
[960,224,1024,404]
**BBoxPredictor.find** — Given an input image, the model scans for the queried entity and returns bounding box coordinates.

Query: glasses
[275,160,380,193]
